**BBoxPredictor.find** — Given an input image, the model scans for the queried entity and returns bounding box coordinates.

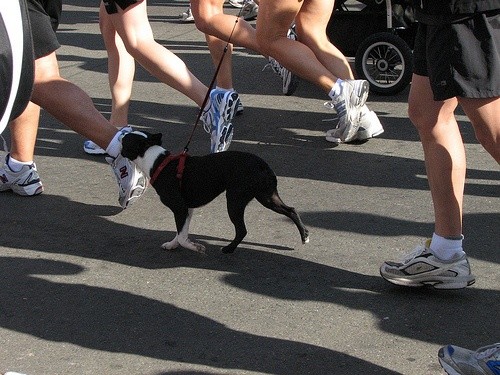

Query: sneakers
[325,110,385,143]
[323,79,370,143]
[439,344,500,375]
[83,124,133,154]
[106,129,149,210]
[380,241,477,290]
[237,101,244,112]
[0,153,43,196]
[177,0,298,42]
[262,56,299,96]
[199,88,241,153]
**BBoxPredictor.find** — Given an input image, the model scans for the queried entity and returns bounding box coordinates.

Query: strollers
[286,0,420,97]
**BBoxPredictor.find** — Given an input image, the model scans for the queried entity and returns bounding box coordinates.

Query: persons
[178,0,384,143]
[84,0,240,155]
[0,0,151,209]
[379,0,500,289]
[438,341,500,375]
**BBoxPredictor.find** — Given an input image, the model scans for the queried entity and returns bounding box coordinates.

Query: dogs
[118,130,310,254]
